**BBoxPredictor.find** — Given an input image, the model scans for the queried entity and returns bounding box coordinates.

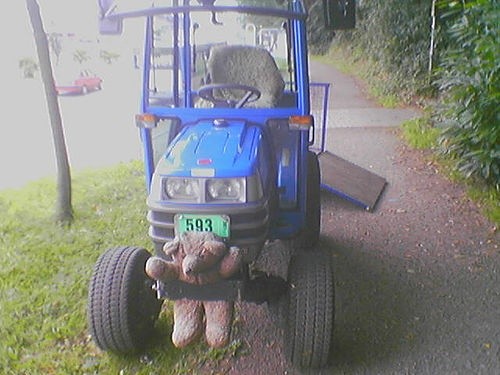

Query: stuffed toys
[146,233,241,348]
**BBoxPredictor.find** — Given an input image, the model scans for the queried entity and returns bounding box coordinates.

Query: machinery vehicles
[89,0,337,375]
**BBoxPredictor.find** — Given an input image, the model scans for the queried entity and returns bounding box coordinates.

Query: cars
[53,70,103,95]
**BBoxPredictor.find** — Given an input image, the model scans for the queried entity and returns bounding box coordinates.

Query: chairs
[203,41,287,109]
